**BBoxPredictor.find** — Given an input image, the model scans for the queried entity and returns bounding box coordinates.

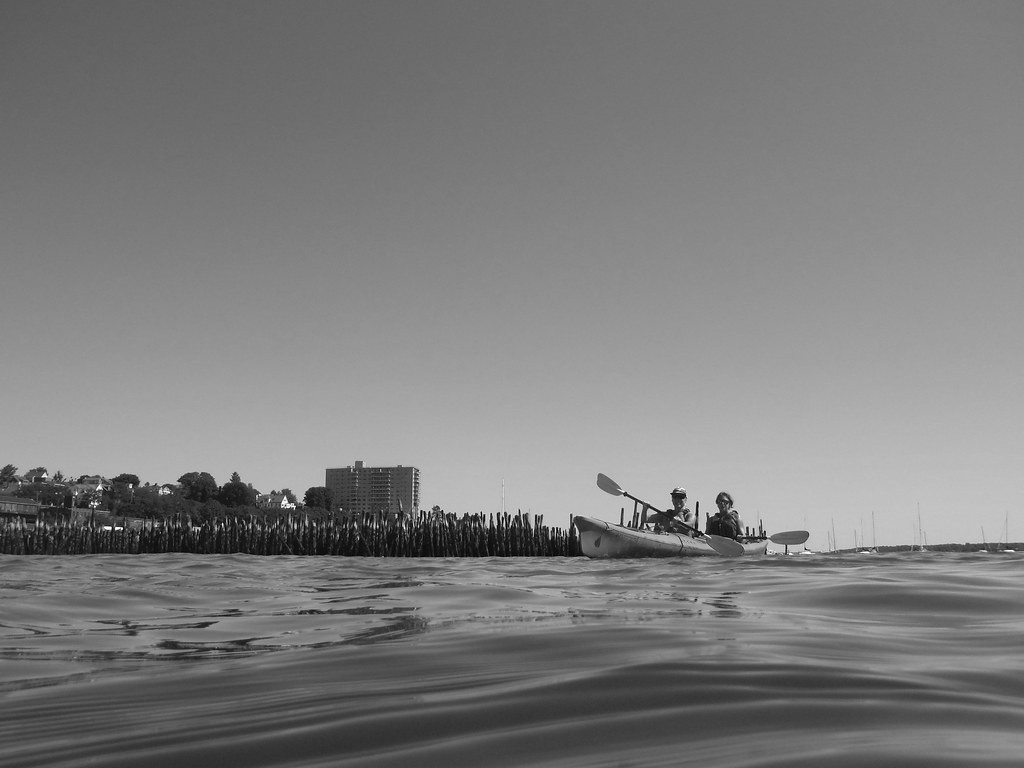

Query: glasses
[672,494,686,499]
[716,499,730,505]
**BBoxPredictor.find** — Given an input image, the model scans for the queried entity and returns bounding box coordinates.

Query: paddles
[596,472,745,558]
[736,530,811,545]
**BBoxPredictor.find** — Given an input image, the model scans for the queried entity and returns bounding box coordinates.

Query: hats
[670,487,686,496]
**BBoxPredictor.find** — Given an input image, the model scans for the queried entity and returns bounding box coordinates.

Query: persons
[641,488,693,537]
[704,493,745,543]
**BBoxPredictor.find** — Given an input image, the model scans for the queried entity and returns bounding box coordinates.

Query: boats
[574,515,769,559]
